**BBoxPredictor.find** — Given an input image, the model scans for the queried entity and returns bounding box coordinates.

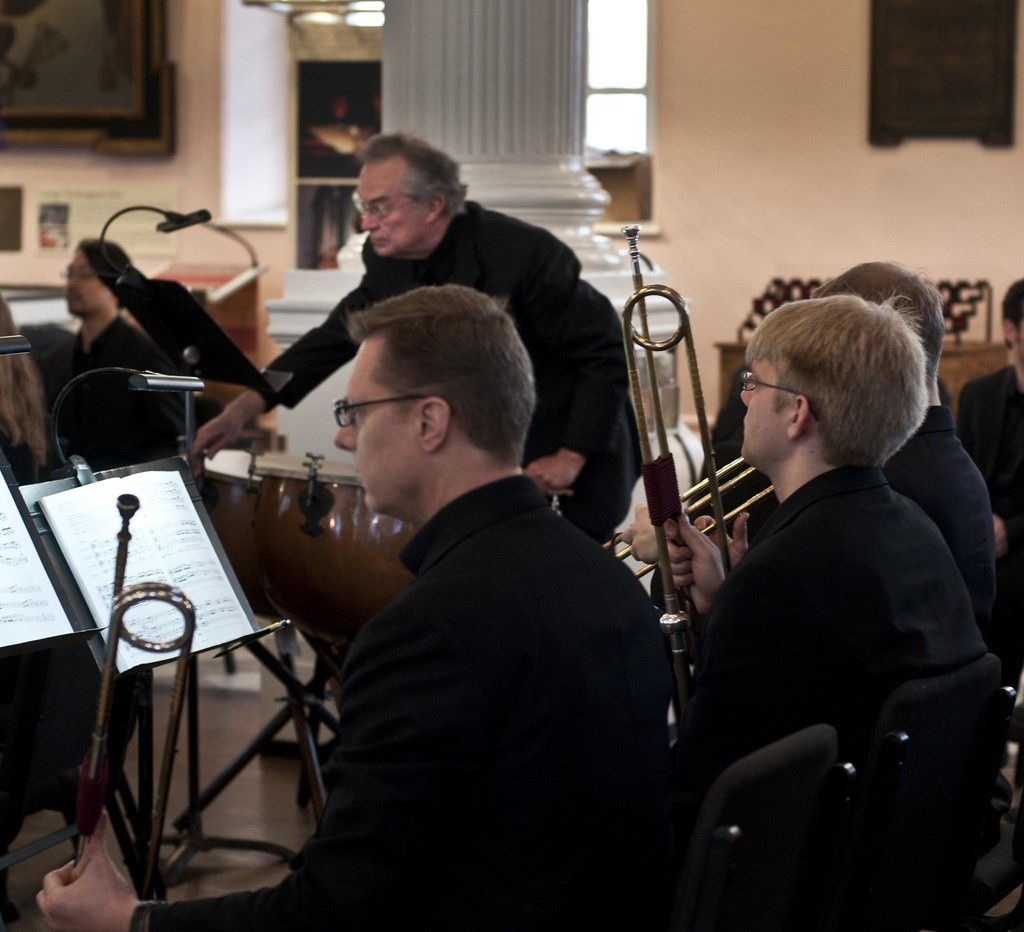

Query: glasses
[740,369,819,422]
[334,398,454,426]
[351,188,445,218]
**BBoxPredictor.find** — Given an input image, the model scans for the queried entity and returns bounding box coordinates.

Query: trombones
[76,493,198,899]
[619,225,734,727]
[600,455,777,578]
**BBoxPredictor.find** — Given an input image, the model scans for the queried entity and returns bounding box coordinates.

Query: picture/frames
[0,0,177,159]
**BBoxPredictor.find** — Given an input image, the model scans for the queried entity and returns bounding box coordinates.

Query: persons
[35,285,676,932]
[192,134,643,549]
[0,240,193,487]
[626,264,1024,932]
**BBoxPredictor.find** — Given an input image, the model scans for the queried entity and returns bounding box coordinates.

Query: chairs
[645,654,1024,932]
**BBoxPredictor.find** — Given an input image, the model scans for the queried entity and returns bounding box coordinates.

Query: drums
[249,460,420,639]
[180,449,301,619]
